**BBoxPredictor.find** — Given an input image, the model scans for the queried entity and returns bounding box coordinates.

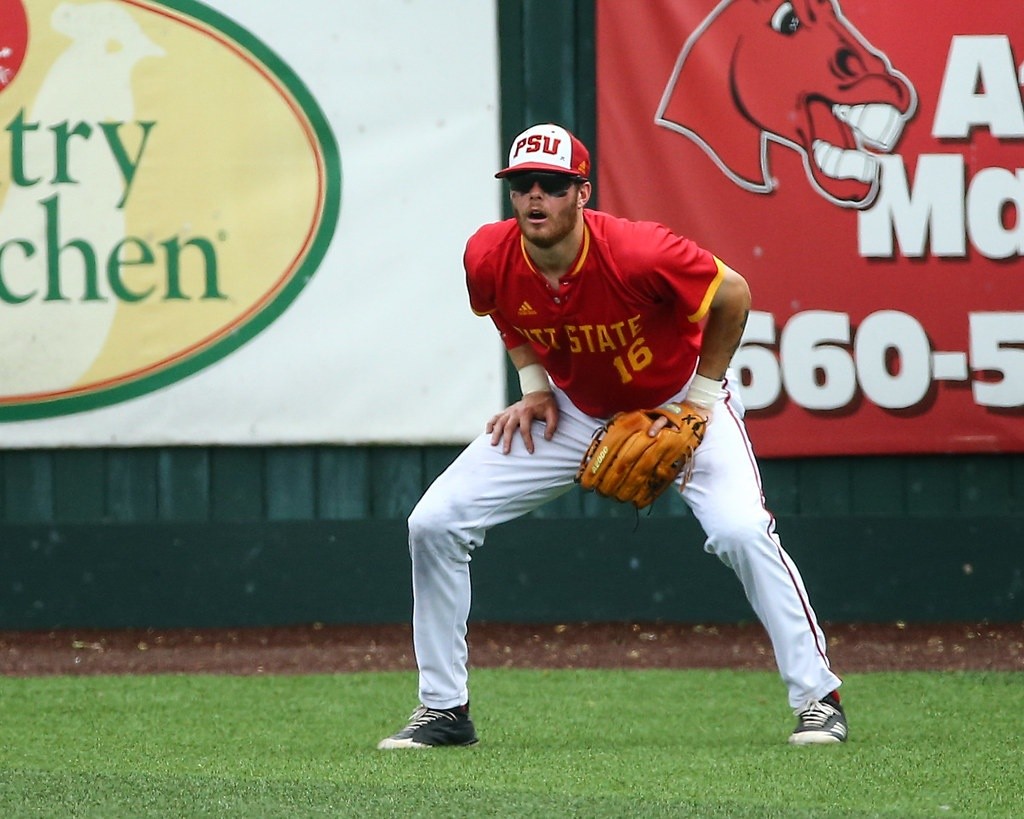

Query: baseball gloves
[569,400,710,534]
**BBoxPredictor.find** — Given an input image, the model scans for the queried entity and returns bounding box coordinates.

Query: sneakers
[377,700,479,749]
[787,694,847,744]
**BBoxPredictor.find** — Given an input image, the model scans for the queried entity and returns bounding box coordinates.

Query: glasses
[510,172,582,192]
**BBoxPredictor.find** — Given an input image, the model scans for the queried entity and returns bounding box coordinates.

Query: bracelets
[518,364,550,395]
[686,375,724,409]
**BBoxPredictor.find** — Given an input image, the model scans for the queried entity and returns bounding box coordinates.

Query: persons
[378,123,848,746]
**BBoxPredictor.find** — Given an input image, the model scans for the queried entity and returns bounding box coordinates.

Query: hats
[495,124,590,179]
